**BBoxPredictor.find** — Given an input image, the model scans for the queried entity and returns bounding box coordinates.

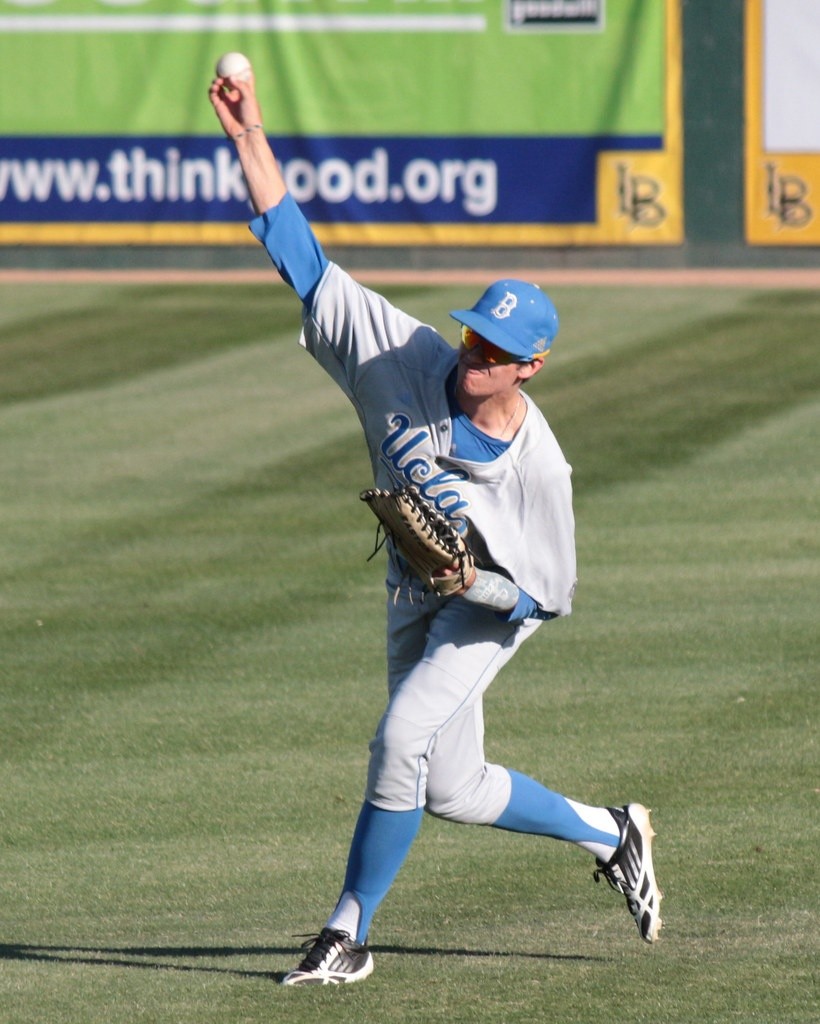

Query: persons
[208,60,664,990]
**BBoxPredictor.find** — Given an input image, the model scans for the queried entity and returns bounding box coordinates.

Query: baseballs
[217,52,251,90]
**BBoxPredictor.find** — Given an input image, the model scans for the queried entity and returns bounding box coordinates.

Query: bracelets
[232,124,261,141]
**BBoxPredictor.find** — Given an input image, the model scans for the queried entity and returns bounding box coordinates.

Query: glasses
[461,323,534,365]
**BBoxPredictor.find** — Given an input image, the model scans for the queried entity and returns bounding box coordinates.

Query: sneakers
[282,927,374,987]
[593,803,662,944]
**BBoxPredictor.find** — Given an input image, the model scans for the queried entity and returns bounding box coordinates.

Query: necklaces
[499,401,521,437]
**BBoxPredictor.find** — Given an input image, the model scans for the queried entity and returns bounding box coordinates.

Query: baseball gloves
[359,486,474,597]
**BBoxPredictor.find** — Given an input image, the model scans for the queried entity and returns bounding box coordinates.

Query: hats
[449,279,558,362]
